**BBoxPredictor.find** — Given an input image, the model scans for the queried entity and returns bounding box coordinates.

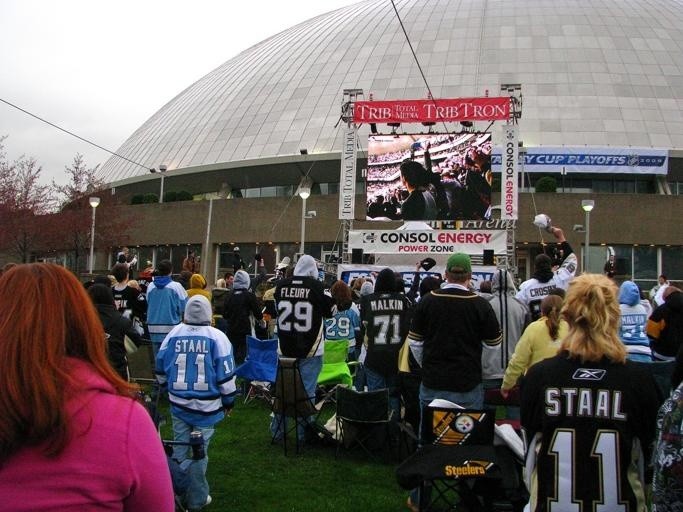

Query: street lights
[296,185,317,261]
[89,195,101,273]
[574,200,594,273]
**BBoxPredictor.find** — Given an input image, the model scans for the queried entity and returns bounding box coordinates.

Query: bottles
[191,431,205,461]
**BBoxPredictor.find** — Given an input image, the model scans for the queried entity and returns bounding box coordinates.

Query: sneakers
[406,495,418,511]
[204,494,212,505]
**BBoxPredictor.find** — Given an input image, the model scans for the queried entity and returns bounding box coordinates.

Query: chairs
[124,340,168,411]
[233,335,401,465]
[418,402,496,512]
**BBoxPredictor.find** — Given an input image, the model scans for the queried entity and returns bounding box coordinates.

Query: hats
[446,253,471,279]
[533,213,553,233]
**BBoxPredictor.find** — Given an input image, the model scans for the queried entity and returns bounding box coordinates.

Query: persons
[365,134,491,221]
[77,226,682,512]
[0,259,178,512]
